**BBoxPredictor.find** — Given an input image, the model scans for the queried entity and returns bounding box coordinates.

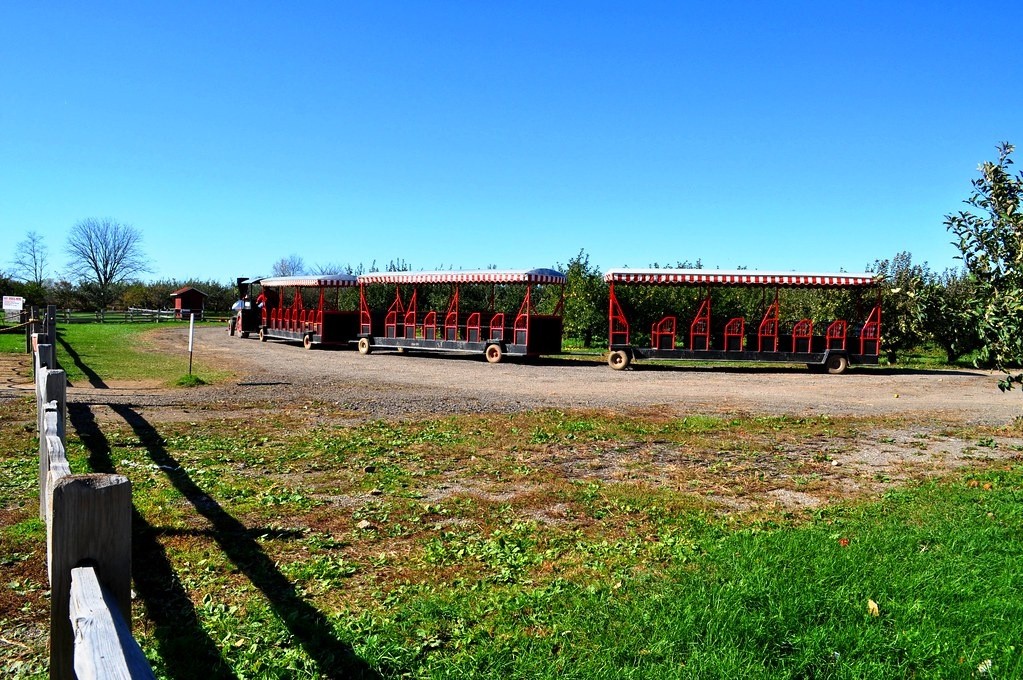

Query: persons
[256,286,280,308]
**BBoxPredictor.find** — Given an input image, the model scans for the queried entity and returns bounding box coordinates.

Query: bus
[226,267,885,374]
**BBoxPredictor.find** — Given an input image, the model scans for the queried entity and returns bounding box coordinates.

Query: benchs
[271,306,880,355]
